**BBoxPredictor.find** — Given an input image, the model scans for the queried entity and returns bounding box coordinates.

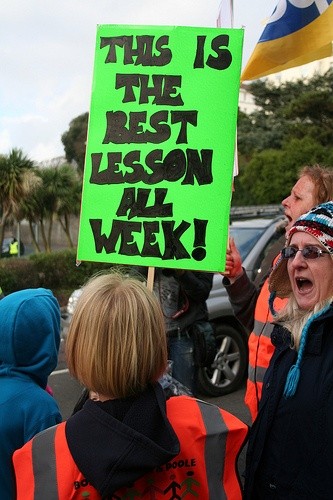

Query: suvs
[66,205,282,399]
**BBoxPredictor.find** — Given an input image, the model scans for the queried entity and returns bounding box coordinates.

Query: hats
[268,200,333,300]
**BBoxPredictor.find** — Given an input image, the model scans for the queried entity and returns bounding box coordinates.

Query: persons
[0,288,64,500]
[118,265,214,398]
[220,163,333,427]
[245,201,333,500]
[6,268,248,500]
[9,237,19,256]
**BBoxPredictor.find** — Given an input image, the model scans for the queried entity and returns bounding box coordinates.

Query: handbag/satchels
[191,320,216,368]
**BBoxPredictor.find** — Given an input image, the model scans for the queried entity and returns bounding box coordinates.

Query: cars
[0,236,25,258]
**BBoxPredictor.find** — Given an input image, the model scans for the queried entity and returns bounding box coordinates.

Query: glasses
[281,246,328,260]
